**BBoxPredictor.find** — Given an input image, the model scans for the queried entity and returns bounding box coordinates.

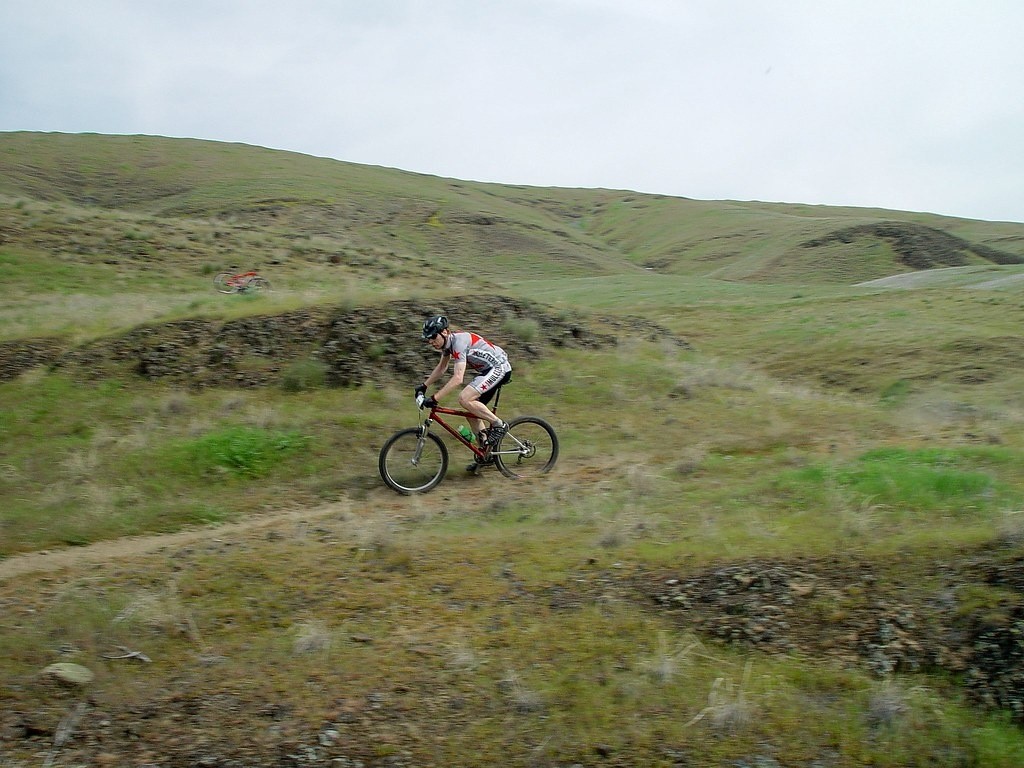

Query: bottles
[458,425,476,444]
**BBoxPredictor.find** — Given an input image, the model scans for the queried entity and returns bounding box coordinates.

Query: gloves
[422,395,438,409]
[414,383,427,399]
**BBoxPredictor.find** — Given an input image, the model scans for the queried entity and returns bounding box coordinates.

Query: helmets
[421,315,448,339]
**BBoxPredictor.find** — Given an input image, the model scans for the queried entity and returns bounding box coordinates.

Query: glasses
[427,332,440,340]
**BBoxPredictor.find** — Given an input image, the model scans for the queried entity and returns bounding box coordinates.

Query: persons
[415,316,512,470]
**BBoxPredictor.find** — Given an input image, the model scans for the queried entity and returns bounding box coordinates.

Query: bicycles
[380,380,559,496]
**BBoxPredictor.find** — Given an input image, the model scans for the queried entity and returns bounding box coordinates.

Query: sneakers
[485,420,509,446]
[466,460,484,472]
